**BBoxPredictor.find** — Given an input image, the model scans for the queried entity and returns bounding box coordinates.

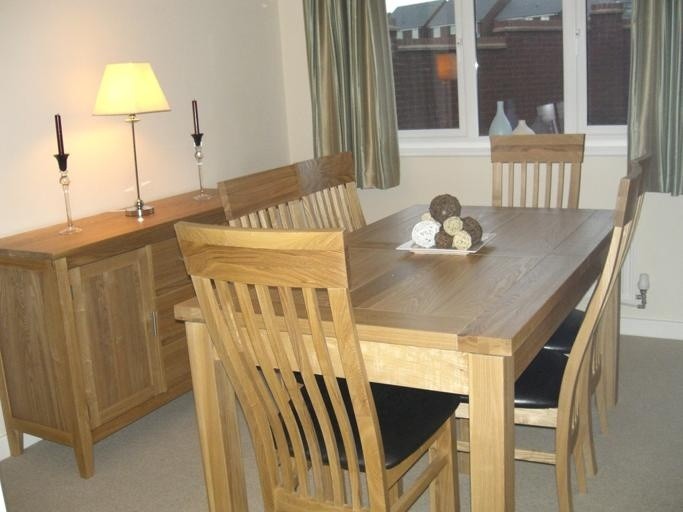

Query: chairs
[486,135,586,210]
[540,153,655,475]
[217,164,310,231]
[174,221,460,510]
[295,151,366,239]
[454,172,642,512]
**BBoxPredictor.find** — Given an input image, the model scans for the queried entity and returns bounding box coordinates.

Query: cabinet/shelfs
[0,189,228,480]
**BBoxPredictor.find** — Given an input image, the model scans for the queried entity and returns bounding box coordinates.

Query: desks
[174,202,623,510]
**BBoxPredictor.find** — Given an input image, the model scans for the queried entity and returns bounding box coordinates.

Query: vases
[487,99,536,136]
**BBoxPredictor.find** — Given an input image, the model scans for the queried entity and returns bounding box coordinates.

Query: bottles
[488,100,535,135]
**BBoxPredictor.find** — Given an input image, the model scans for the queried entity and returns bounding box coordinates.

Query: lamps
[90,61,173,218]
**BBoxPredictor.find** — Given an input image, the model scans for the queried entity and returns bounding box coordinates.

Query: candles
[191,100,199,134]
[53,113,64,156]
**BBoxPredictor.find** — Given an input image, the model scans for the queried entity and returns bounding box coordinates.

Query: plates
[396,230,497,257]
